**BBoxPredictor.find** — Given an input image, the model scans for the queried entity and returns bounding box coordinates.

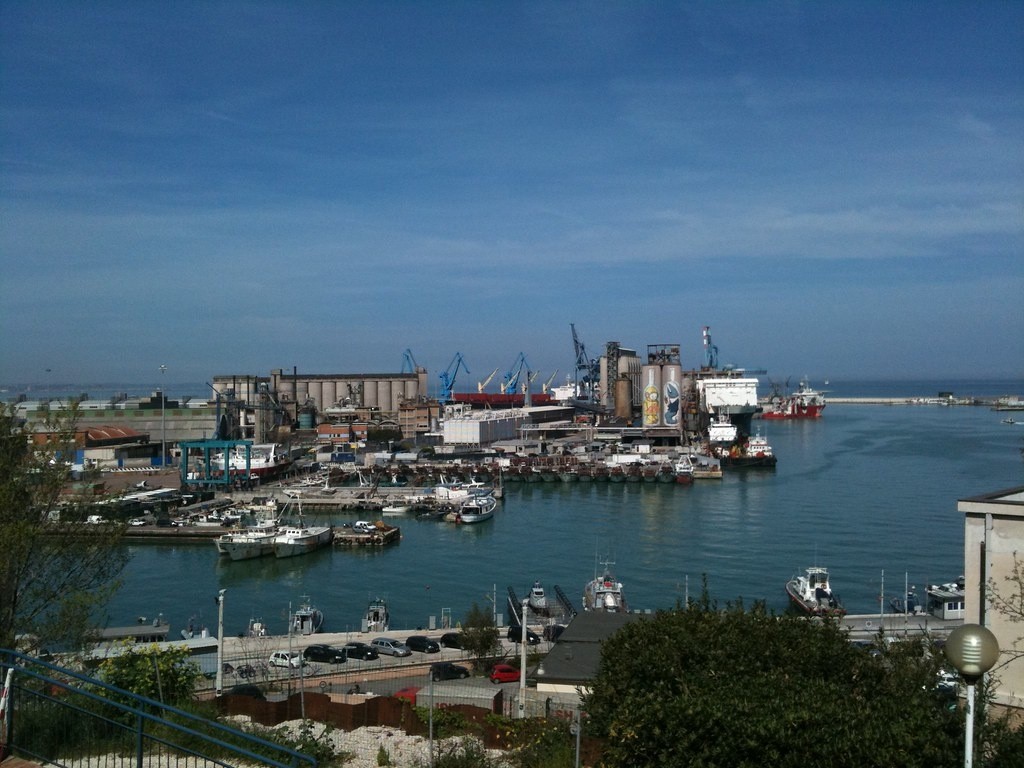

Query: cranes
[542,369,559,394]
[522,369,540,394]
[401,349,417,373]
[438,351,470,398]
[478,367,499,394]
[568,323,592,400]
[504,352,532,394]
[501,371,520,395]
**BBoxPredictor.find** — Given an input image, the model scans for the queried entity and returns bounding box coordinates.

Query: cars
[507,625,541,645]
[406,635,440,654]
[853,640,881,656]
[371,637,411,658]
[342,642,378,661]
[127,519,146,526]
[204,663,234,679]
[352,525,371,534]
[303,644,346,664]
[356,521,376,530]
[269,650,305,668]
[232,665,256,679]
[489,664,521,685]
[88,515,109,523]
[429,662,470,682]
[441,632,472,651]
[543,625,566,643]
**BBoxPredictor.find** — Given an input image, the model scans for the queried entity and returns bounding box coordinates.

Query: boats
[526,580,551,619]
[248,617,268,636]
[674,455,695,485]
[626,465,643,482]
[460,489,496,523]
[219,509,280,561]
[657,461,675,483]
[583,558,629,613]
[381,505,412,513]
[358,462,609,483]
[888,586,923,613]
[213,534,241,555]
[609,466,626,482]
[785,567,847,617]
[272,514,332,559]
[211,443,295,481]
[366,596,390,633]
[293,601,324,635]
[643,465,657,483]
[707,407,777,468]
[761,375,827,419]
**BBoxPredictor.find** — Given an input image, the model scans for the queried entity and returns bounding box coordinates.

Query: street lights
[945,624,1000,767]
[158,364,167,467]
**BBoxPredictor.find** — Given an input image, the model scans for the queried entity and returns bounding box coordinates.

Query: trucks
[395,685,504,716]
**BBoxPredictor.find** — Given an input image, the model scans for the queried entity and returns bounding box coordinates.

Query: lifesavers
[333,538,381,545]
